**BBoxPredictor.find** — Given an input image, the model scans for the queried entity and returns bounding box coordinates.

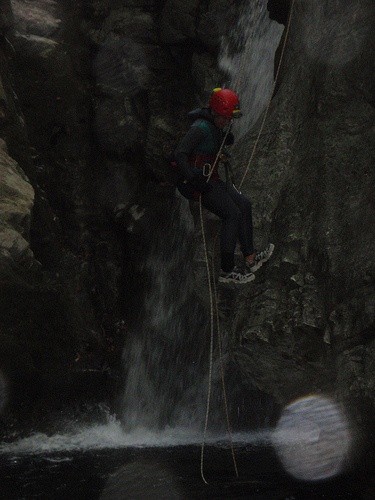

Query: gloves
[195,179,211,193]
[225,132,234,145]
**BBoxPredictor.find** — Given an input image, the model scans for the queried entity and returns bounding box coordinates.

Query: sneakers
[218,266,255,284]
[248,243,275,273]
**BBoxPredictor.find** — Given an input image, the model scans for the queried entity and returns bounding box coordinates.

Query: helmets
[209,87,241,119]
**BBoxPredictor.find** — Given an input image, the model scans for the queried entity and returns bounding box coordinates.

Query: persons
[173,89,274,284]
